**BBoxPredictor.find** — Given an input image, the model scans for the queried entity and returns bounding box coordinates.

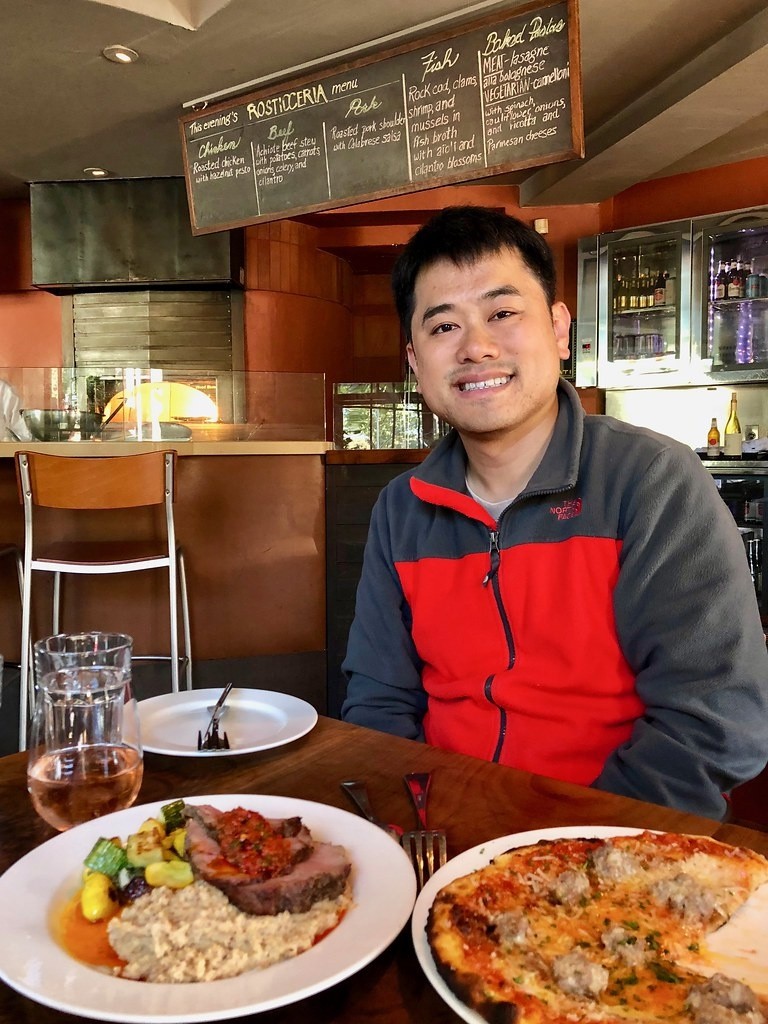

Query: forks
[401,773,447,889]
[198,682,234,752]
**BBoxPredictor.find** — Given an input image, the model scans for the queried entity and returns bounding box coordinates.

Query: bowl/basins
[18,408,106,442]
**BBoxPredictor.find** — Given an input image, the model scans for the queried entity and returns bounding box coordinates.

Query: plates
[412,826,667,1024]
[134,422,192,442]
[121,688,318,757]
[0,794,417,1024]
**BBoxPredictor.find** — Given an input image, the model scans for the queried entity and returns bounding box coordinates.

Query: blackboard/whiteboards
[177,0,586,238]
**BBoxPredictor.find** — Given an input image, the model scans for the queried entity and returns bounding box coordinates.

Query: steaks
[188,814,351,909]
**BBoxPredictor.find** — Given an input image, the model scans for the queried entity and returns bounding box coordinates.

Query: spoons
[340,780,400,845]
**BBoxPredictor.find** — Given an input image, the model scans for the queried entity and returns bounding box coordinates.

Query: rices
[106,881,350,981]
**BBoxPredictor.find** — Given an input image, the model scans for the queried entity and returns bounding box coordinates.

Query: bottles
[724,393,742,459]
[707,418,720,456]
[613,267,669,311]
[714,260,752,300]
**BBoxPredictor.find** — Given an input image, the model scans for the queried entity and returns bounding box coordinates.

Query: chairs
[12,449,192,752]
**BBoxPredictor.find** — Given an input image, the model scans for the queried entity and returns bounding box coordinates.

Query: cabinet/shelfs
[597,207,768,388]
[705,468,768,653]
[326,466,419,722]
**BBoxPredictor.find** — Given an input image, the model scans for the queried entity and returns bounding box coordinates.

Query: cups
[30,632,134,753]
[27,666,144,832]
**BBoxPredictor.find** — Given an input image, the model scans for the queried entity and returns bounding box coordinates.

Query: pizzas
[428,830,767,1023]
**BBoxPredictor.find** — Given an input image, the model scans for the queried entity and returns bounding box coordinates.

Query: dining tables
[0,711,767,1024]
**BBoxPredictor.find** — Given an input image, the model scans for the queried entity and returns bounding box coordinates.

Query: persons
[341,206,768,819]
[0,379,33,443]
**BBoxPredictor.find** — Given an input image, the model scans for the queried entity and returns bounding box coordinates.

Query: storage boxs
[744,495,765,523]
[719,480,764,523]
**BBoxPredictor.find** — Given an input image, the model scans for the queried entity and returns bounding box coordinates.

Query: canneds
[746,274,761,298]
[614,334,659,354]
